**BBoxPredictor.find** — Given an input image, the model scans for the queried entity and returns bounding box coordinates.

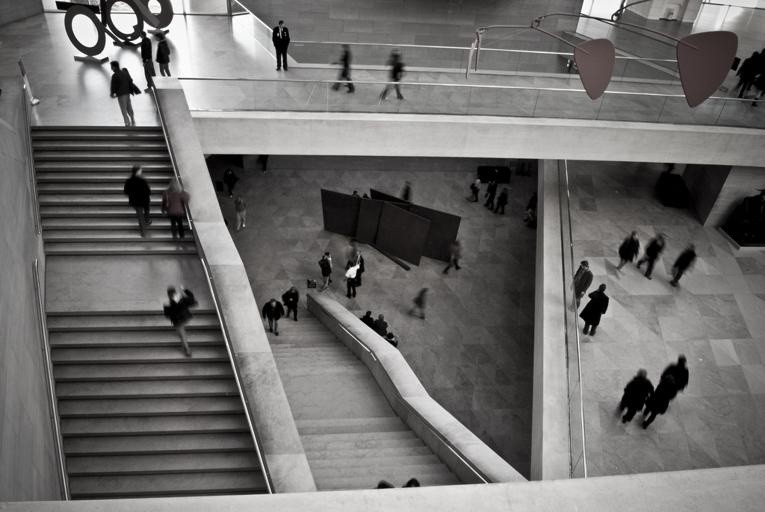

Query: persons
[272,20,290,71]
[619,355,689,430]
[110,61,135,127]
[668,242,696,284]
[328,44,355,93]
[401,181,410,202]
[282,286,298,321]
[318,248,365,299]
[160,181,189,241]
[380,48,405,100]
[579,283,609,336]
[471,175,508,215]
[363,194,369,199]
[123,166,152,238]
[352,191,359,197]
[523,192,537,226]
[211,167,247,231]
[443,237,461,275]
[635,233,664,280]
[616,230,640,271]
[162,285,195,357]
[361,311,398,346]
[409,288,428,320]
[155,32,171,77]
[574,260,593,309]
[139,31,153,91]
[734,46,765,107]
[262,299,284,336]
